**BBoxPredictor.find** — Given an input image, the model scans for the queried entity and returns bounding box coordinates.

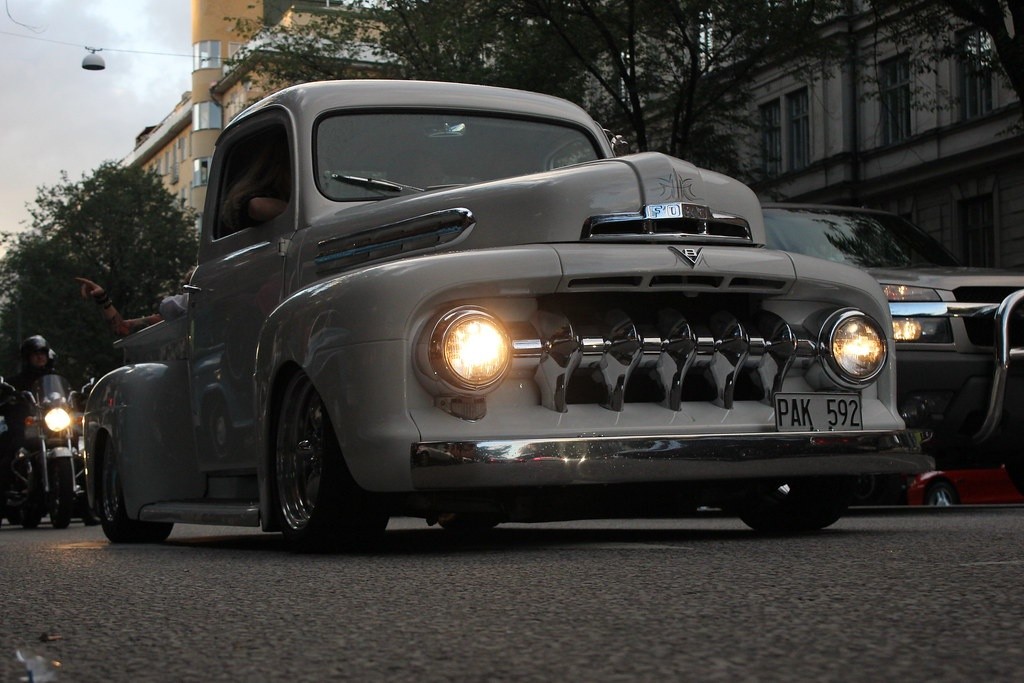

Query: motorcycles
[0,377,99,529]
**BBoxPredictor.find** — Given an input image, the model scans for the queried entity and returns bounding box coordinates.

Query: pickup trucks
[82,78,909,550]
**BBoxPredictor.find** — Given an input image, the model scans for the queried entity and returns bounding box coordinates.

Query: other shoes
[83,514,100,526]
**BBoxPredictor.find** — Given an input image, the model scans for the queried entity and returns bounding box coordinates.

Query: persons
[0,335,102,530]
[248,148,331,226]
[76,267,202,337]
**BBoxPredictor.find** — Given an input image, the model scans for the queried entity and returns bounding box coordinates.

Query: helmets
[45,348,57,373]
[20,334,49,368]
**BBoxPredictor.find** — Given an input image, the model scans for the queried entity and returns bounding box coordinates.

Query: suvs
[764,204,1023,496]
